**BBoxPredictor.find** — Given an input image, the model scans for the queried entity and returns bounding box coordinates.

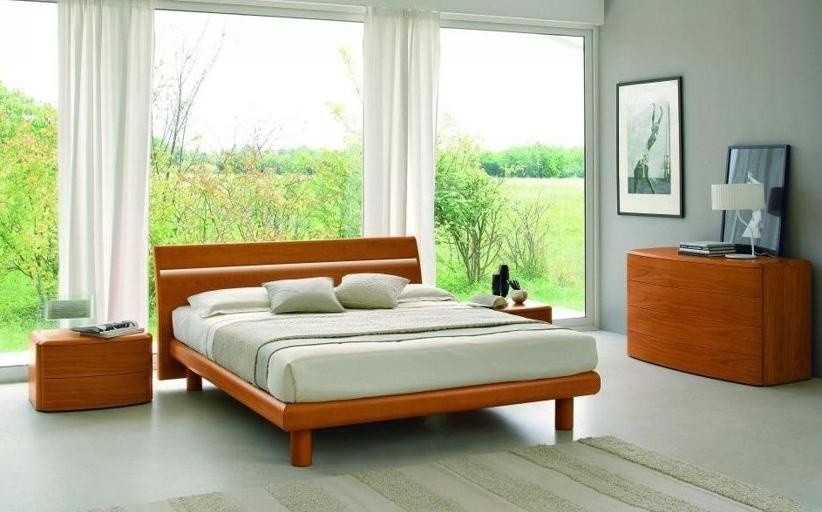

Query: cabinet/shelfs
[624,245,814,388]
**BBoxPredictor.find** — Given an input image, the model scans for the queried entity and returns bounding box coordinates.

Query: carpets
[83,432,811,511]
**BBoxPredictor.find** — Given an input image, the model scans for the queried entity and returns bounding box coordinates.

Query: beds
[150,235,604,473]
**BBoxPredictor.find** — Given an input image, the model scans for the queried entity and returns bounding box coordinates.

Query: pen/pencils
[506,278,521,289]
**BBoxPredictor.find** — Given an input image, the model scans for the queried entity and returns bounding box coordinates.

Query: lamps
[710,181,767,260]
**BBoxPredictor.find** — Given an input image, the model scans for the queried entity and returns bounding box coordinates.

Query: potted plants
[508,280,529,304]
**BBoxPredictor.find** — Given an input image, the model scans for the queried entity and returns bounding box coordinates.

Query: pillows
[184,269,459,320]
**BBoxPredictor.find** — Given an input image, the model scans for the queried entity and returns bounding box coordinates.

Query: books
[79,327,146,339]
[71,319,140,333]
[677,240,736,258]
[465,292,509,308]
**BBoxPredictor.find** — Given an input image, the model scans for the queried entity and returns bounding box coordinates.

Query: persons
[742,163,767,246]
[632,102,663,194]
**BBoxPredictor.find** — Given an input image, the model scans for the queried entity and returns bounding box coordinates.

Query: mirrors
[718,144,792,256]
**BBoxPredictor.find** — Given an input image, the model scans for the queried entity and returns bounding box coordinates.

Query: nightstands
[26,329,154,413]
[483,297,554,323]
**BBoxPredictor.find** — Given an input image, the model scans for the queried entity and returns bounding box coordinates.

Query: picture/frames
[613,75,687,222]
